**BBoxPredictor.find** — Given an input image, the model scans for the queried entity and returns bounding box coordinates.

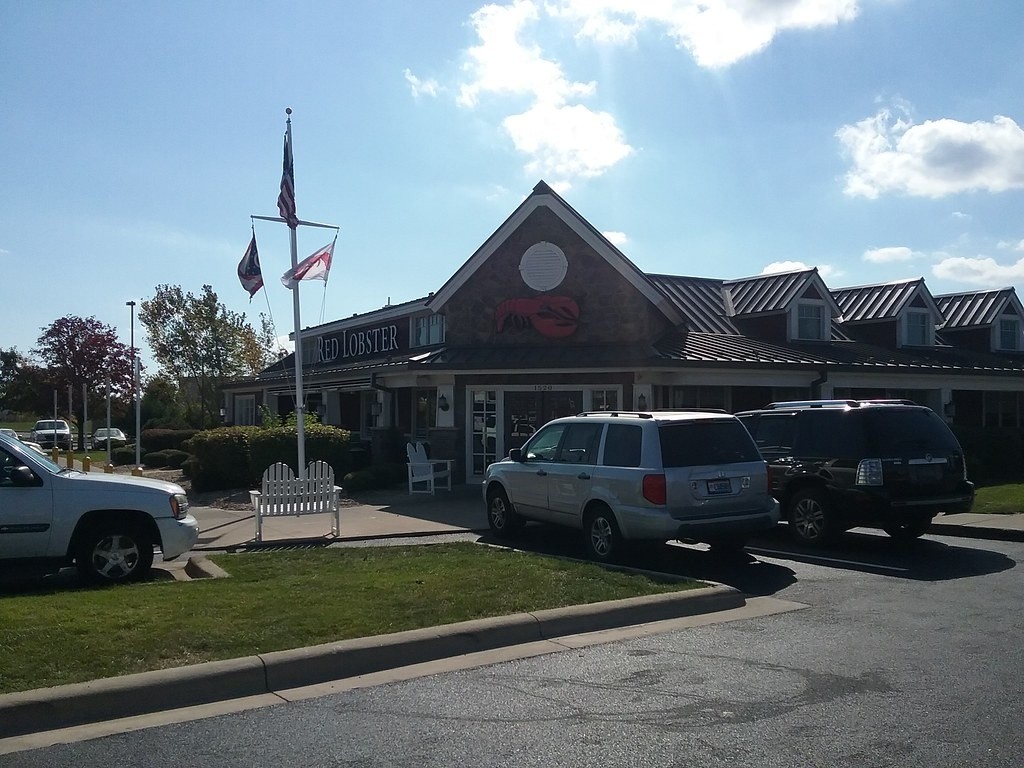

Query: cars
[0,428,48,458]
[90,427,126,450]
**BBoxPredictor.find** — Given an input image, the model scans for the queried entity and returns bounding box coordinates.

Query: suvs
[480,408,782,561]
[733,397,978,549]
[29,420,74,451]
[0,430,200,585]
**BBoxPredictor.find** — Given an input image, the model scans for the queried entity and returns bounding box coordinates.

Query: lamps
[944,400,955,417]
[371,402,382,416]
[220,407,226,417]
[316,405,326,417]
[438,394,449,411]
[638,393,648,411]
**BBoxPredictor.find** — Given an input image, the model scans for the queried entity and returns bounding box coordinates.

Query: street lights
[125,301,135,425]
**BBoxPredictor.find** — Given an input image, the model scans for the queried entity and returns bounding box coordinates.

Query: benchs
[406,441,455,496]
[249,460,343,541]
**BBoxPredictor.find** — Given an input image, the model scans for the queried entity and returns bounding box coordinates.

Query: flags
[276,135,299,230]
[280,240,335,290]
[237,235,264,304]
